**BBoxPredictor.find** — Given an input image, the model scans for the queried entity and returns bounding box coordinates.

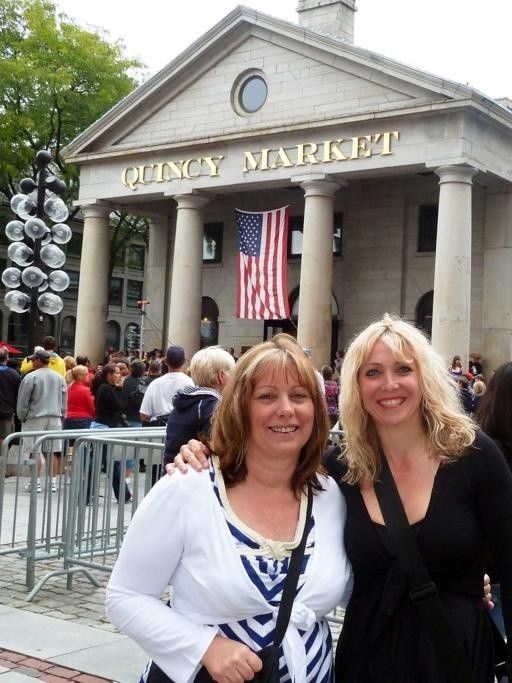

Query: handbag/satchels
[144,642,282,682]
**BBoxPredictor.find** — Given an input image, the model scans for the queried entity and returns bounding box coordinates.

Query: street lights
[2,148,71,358]
[124,323,140,355]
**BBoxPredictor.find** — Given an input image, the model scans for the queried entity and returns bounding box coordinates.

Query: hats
[28,350,51,364]
[470,353,481,359]
[458,374,469,388]
[167,346,184,365]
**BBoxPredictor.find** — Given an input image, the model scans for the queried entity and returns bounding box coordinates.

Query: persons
[447,352,487,417]
[0,335,196,507]
[320,365,339,430]
[102,332,495,683]
[471,362,512,471]
[333,350,344,384]
[228,347,238,363]
[164,312,511,683]
[162,345,236,480]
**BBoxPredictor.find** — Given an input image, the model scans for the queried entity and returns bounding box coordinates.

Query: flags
[233,205,292,321]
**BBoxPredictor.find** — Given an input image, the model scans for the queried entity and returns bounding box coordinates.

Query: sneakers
[25,481,41,492]
